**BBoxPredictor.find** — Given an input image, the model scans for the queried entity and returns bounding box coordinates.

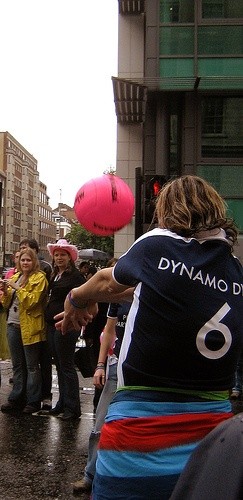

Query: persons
[68,300,132,494]
[164,358,243,500]
[52,175,243,500]
[0,236,117,421]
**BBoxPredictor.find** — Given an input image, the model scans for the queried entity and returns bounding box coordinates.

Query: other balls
[75,175,135,235]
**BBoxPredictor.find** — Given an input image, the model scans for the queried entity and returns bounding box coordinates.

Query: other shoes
[49,407,64,415]
[62,414,72,419]
[40,404,52,412]
[9,378,14,383]
[23,403,41,413]
[1,401,25,411]
[74,480,91,491]
[231,392,241,398]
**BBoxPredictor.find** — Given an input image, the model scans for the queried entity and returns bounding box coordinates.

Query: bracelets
[92,361,106,374]
[68,290,89,309]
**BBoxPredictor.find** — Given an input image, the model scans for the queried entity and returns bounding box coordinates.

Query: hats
[47,239,78,262]
